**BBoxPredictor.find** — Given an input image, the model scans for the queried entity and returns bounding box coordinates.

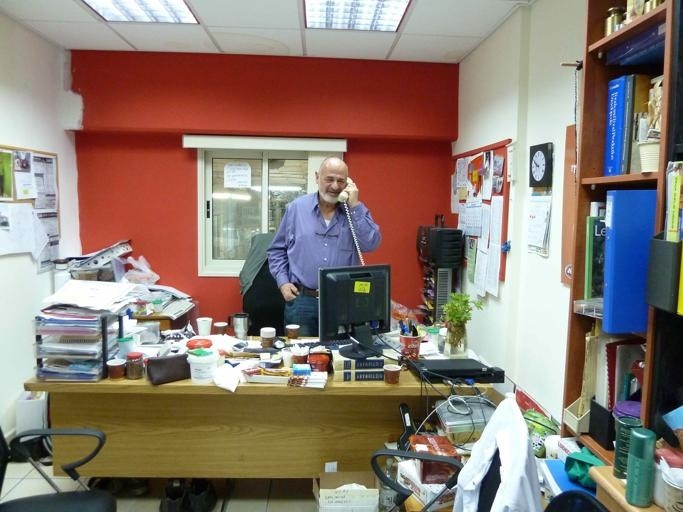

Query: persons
[267,154,382,339]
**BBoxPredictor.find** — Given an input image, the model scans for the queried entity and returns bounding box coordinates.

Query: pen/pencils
[399,317,422,336]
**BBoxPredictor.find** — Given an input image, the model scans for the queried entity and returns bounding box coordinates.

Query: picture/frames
[0,145,61,257]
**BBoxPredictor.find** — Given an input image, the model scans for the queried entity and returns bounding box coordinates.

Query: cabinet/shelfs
[556,0,683,467]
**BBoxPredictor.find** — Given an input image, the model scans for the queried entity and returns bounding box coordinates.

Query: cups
[260,327,276,348]
[196,314,250,342]
[107,360,126,379]
[400,335,419,362]
[285,325,300,340]
[661,468,683,511]
[383,365,400,384]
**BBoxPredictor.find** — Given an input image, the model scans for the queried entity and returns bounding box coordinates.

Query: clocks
[528,143,553,188]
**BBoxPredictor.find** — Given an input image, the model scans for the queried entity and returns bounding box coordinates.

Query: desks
[24,335,493,499]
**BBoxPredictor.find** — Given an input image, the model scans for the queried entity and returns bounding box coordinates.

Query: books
[575,154,683,473]
[33,276,150,381]
[330,349,384,384]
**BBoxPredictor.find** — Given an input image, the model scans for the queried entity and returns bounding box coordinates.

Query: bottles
[626,426,654,509]
[427,328,449,353]
[118,337,144,380]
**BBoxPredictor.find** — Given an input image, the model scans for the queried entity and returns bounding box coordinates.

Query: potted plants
[439,291,482,354]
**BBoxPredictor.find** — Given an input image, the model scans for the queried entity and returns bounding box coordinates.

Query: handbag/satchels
[147,354,191,385]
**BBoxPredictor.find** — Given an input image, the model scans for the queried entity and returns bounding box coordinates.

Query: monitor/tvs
[318,264,390,359]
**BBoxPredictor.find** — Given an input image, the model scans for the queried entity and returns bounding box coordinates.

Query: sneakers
[122,477,150,496]
[158,478,218,512]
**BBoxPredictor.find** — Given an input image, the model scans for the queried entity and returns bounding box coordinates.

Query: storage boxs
[312,471,379,512]
[398,460,457,512]
[434,400,496,445]
[409,434,463,484]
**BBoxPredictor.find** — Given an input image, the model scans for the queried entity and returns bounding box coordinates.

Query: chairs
[0,426,118,512]
[369,398,531,512]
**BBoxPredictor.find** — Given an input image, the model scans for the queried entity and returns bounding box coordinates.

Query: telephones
[337,176,354,203]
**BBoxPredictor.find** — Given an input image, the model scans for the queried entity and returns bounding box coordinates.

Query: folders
[601,190,657,333]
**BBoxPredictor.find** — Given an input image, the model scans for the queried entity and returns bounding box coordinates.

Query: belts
[296,285,319,298]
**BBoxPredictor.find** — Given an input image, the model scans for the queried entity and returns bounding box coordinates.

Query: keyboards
[296,339,354,350]
